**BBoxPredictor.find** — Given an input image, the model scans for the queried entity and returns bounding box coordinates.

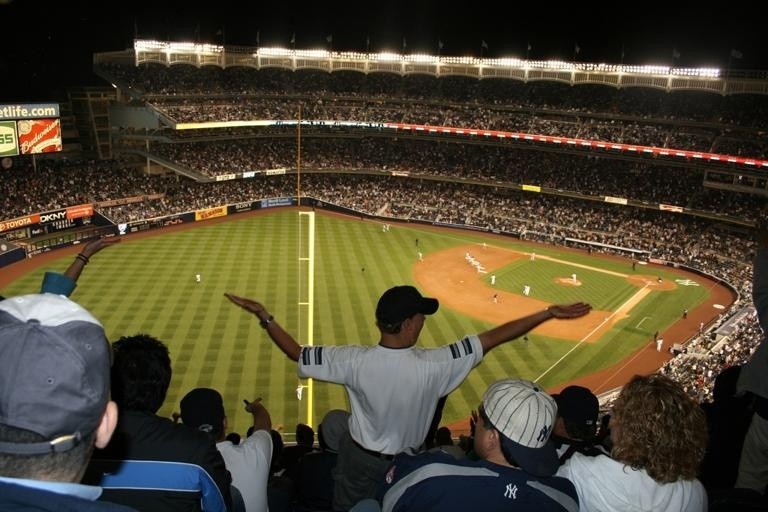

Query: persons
[0,60,765,512]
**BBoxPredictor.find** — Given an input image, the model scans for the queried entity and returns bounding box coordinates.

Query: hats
[373,284,441,325]
[554,384,603,438]
[176,387,227,440]
[479,377,562,477]
[1,291,115,454]
[321,407,351,451]
